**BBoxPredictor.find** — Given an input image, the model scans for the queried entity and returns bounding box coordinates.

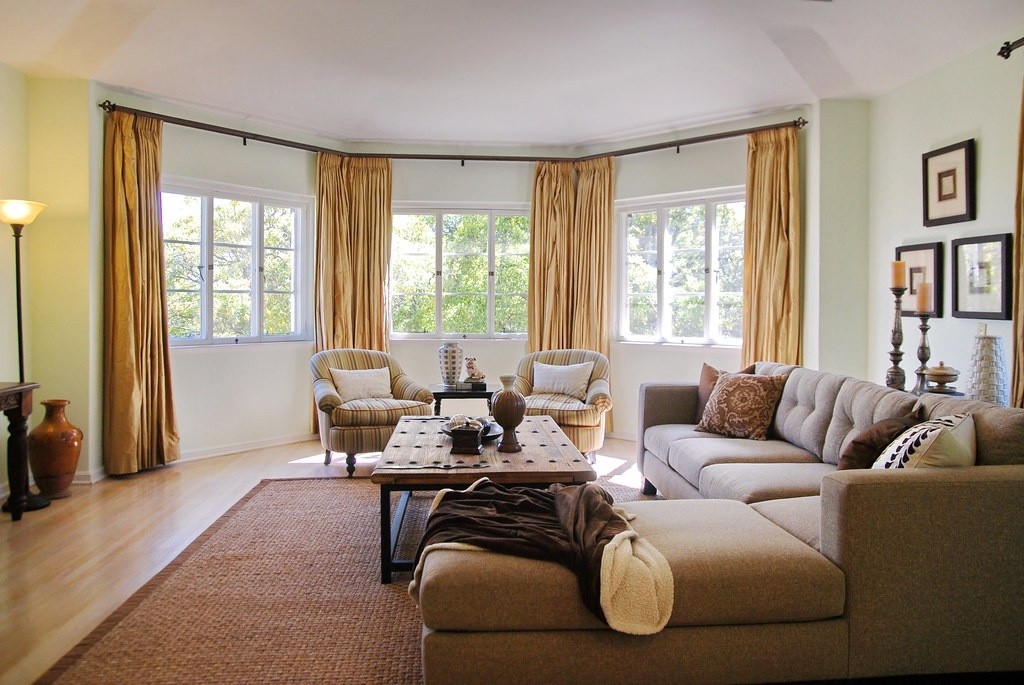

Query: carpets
[34,479,669,685]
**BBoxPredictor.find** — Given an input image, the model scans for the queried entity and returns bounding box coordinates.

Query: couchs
[422,360,1024,685]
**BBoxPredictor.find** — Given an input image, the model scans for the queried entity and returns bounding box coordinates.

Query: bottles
[492,374,526,453]
[28,399,84,500]
[438,341,463,389]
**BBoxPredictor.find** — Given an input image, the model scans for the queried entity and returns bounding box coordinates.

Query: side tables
[428,382,503,417]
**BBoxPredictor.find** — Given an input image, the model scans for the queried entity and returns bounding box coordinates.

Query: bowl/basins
[440,422,504,444]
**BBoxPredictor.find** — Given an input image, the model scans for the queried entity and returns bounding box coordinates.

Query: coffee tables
[370,415,597,584]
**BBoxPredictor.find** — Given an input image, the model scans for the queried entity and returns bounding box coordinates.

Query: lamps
[0,199,52,512]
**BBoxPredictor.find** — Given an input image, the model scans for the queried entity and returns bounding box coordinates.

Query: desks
[0,381,40,522]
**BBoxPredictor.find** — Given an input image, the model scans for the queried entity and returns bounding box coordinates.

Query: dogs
[464,357,485,379]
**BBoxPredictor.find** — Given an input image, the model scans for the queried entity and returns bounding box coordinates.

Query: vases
[30,400,83,499]
[965,334,1008,408]
[438,342,464,388]
[492,376,526,453]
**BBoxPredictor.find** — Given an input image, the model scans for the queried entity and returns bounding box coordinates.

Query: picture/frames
[896,242,944,319]
[952,233,1013,321]
[922,138,977,228]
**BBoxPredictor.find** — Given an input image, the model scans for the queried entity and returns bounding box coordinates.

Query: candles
[890,261,905,288]
[916,282,932,313]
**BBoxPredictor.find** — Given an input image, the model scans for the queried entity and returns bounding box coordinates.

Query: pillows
[872,411,977,470]
[837,402,923,471]
[695,362,755,423]
[328,366,393,403]
[694,370,788,440]
[533,361,593,401]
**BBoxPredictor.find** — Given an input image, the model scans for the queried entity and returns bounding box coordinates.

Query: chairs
[492,349,613,465]
[310,349,435,478]
